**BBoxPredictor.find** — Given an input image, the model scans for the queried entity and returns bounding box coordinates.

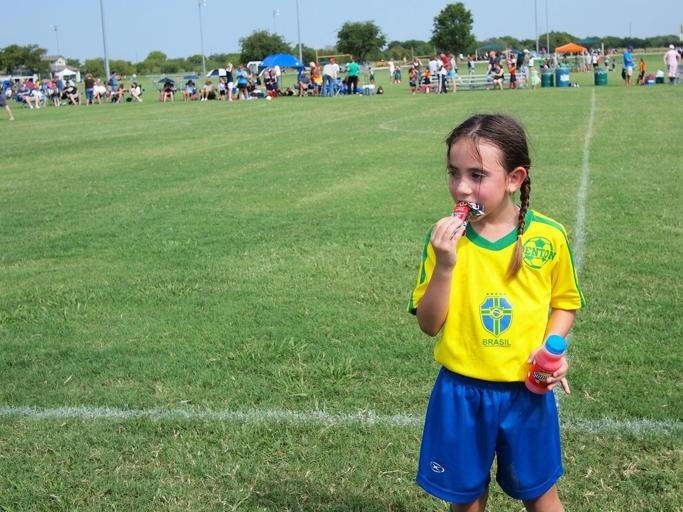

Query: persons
[1,40,682,121]
[405,114,588,512]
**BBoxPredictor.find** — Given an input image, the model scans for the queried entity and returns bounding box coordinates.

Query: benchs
[419,74,527,91]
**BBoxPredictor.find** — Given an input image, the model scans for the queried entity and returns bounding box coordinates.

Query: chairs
[334,86,341,96]
[15,83,239,108]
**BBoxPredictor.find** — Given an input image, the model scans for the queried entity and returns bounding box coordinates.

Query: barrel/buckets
[595,66,608,85]
[556,68,569,87]
[656,77,664,83]
[541,73,553,87]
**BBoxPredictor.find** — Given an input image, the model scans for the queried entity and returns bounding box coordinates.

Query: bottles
[524,334,567,394]
